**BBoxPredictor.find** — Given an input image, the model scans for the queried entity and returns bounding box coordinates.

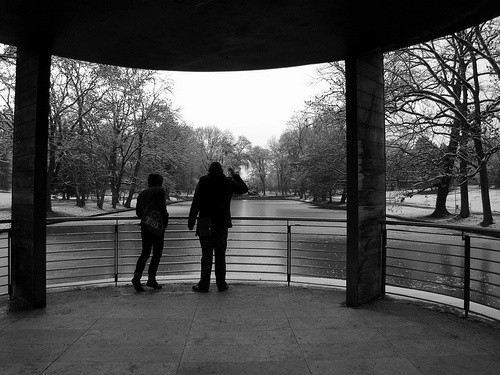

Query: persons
[129,172,171,292]
[186,161,249,292]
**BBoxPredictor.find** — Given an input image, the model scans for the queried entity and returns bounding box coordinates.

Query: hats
[208,162,223,173]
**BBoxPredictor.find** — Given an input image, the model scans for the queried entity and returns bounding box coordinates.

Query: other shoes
[131,278,144,292]
[218,285,228,292]
[145,278,162,289]
[192,285,208,292]
